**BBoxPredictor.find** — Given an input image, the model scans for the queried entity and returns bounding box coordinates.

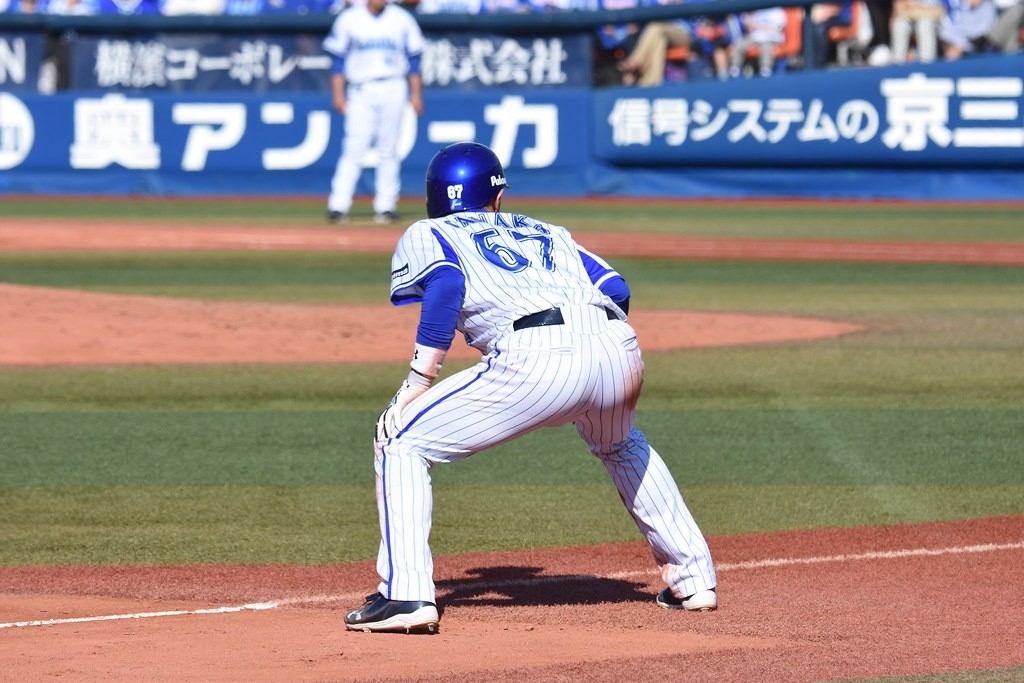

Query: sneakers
[656,586,717,609]
[343,592,439,634]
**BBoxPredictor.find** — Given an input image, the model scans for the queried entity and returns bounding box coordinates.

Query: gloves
[375,370,432,442]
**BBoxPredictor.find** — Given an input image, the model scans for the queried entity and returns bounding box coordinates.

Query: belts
[513,306,619,331]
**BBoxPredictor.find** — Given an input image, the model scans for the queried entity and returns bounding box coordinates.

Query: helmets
[426,143,510,218]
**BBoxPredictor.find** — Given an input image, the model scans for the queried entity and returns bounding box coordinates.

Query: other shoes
[380,211,396,223]
[329,211,346,225]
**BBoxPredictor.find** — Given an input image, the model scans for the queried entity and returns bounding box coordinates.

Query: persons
[616,0,1024,88]
[323,0,427,224]
[344,141,717,635]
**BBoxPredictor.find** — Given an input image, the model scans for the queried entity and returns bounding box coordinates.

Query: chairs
[726,7,803,76]
[827,1,860,43]
[667,46,692,62]
